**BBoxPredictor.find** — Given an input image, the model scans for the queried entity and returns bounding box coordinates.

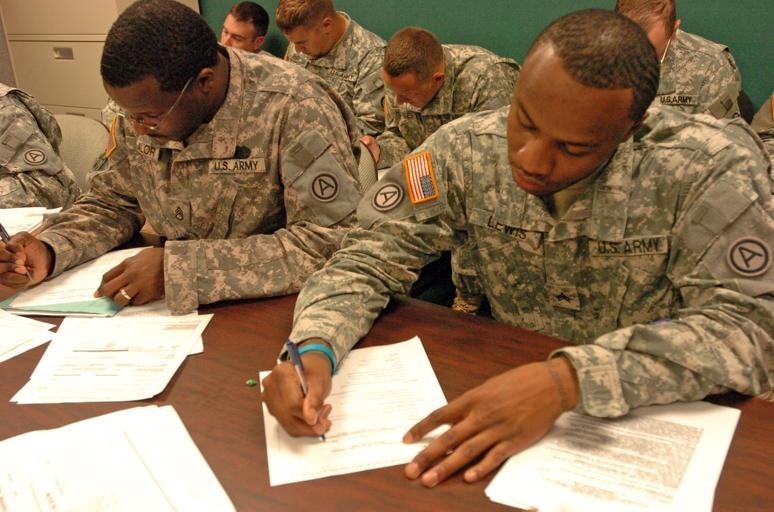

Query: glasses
[105,76,193,132]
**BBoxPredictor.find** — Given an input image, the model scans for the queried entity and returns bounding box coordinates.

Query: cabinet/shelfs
[0,1,200,139]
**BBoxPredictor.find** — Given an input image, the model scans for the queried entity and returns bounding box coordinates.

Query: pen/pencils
[286,339,325,440]
[0,223,33,280]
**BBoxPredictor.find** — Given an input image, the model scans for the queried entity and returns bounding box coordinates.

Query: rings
[119,288,131,300]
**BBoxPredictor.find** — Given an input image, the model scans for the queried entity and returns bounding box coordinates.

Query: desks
[1,276,774,512]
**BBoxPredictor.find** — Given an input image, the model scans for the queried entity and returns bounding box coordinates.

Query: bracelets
[285,343,336,380]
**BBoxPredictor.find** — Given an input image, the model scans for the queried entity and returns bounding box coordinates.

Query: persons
[749,95,773,188]
[274,0,390,136]
[0,0,362,315]
[357,26,519,171]
[214,1,271,56]
[614,1,744,120]
[0,81,81,209]
[251,7,773,488]
